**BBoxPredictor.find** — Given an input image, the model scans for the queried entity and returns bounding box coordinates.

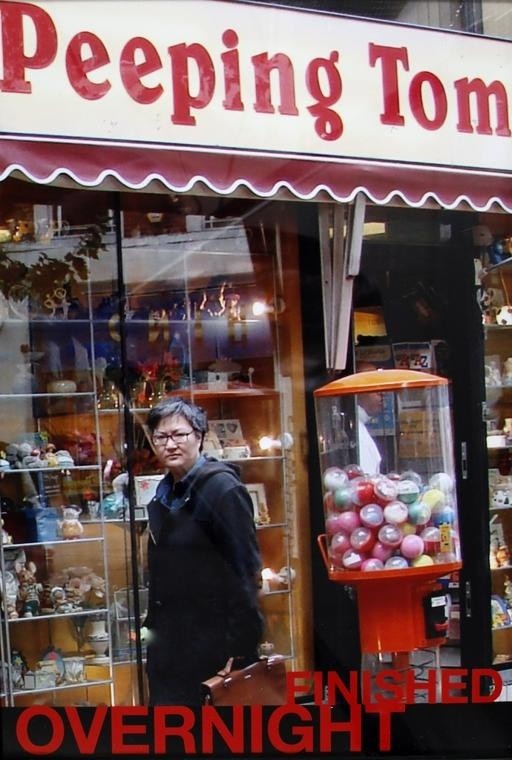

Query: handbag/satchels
[201,654,289,706]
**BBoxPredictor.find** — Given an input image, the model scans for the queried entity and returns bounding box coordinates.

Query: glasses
[147,428,197,448]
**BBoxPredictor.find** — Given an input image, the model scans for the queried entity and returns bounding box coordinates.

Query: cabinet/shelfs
[0,203,315,708]
[447,241,512,671]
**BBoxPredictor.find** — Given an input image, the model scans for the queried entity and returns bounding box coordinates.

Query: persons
[142,394,263,707]
[358,360,387,475]
